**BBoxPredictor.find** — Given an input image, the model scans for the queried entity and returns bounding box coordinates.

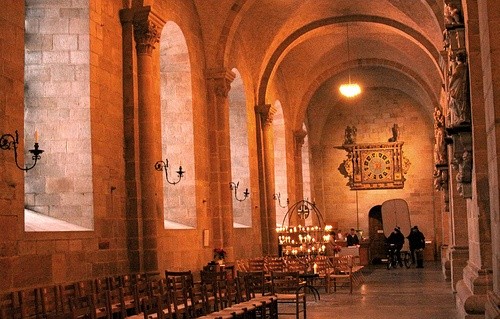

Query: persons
[392,123,401,141]
[434,108,447,164]
[344,124,357,144]
[338,230,343,240]
[408,226,426,269]
[347,228,359,246]
[326,231,339,265]
[448,50,470,124]
[384,226,404,268]
[456,151,472,183]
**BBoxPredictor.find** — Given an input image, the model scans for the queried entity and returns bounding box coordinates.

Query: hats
[412,226,418,230]
[394,227,400,232]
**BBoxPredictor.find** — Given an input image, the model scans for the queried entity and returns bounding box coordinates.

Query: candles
[275,224,333,259]
[179,159,182,168]
[35,129,39,144]
[314,197,316,202]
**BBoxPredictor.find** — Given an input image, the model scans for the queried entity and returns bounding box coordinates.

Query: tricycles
[385,243,412,270]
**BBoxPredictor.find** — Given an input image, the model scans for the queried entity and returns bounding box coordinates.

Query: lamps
[313,262,317,274]
[339,23,362,98]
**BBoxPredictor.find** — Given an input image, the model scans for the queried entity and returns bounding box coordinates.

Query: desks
[291,273,320,303]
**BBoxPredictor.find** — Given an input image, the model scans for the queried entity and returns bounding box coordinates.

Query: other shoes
[421,265,424,268]
[401,264,403,267]
[416,265,420,268]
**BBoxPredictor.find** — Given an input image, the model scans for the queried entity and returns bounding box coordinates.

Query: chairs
[0,253,365,319]
